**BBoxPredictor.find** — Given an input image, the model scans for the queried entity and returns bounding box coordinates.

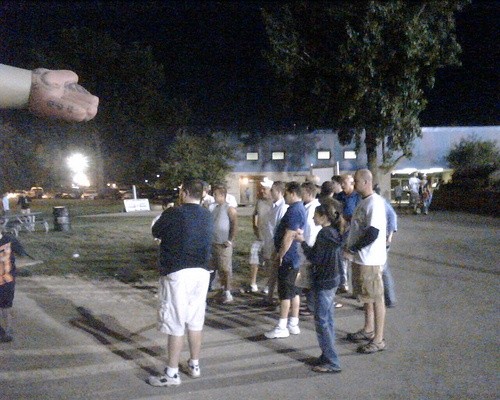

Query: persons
[246,174,397,315]
[343,169,387,354]
[264,181,306,337]
[152,184,238,303]
[294,204,344,371]
[406,172,432,215]
[373,184,381,195]
[0,64,99,122]
[3,194,9,216]
[148,182,213,385]
[18,192,32,214]
[394,183,402,207]
[0,229,25,341]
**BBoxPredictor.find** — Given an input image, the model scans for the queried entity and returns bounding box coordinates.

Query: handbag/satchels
[286,259,314,290]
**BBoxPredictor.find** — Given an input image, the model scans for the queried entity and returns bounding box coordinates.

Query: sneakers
[263,326,290,338]
[182,360,201,378]
[286,324,301,334]
[148,372,181,387]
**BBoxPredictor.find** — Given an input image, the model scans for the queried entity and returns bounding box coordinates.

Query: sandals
[358,339,386,353]
[347,329,374,342]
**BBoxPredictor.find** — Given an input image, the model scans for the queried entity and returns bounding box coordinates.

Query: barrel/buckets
[53,205,73,232]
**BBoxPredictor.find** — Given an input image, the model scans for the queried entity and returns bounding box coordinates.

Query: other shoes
[336,285,349,295]
[264,304,277,310]
[299,308,314,315]
[308,356,329,365]
[251,298,272,307]
[2,332,12,342]
[312,364,338,371]
[207,290,233,303]
[334,302,343,308]
[239,283,258,293]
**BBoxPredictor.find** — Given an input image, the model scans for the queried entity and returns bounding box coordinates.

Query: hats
[260,178,274,187]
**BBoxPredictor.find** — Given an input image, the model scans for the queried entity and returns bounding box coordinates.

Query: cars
[157,186,180,200]
[54,182,82,199]
[96,182,126,199]
[130,186,159,200]
[30,187,44,198]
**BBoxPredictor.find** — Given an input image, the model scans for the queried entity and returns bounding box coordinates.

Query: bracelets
[226,240,232,244]
